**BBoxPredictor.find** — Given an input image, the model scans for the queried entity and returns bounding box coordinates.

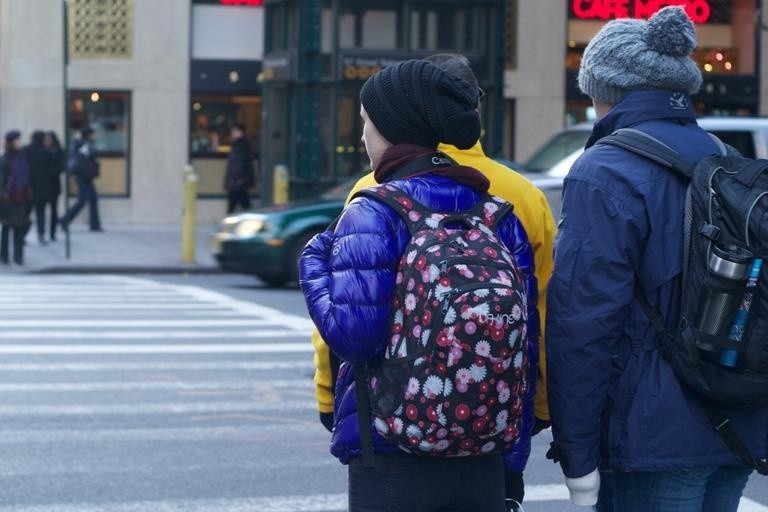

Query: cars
[208,162,374,290]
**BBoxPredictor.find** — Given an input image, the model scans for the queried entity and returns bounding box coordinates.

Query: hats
[359,59,483,151]
[576,5,703,107]
[6,131,20,143]
[81,125,95,138]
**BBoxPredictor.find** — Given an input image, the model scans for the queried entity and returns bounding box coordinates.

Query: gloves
[325,209,347,234]
[530,417,551,436]
[318,412,335,432]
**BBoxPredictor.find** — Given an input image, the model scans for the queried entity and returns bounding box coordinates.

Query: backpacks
[596,127,764,414]
[348,184,530,461]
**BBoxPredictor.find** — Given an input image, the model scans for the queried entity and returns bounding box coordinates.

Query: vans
[514,115,768,226]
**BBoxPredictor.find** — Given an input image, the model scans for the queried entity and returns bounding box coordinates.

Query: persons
[23,130,48,244]
[222,122,256,215]
[0,128,29,266]
[59,127,104,232]
[41,132,66,240]
[544,4,766,511]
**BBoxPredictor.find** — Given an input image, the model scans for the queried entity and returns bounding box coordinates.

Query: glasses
[479,87,486,105]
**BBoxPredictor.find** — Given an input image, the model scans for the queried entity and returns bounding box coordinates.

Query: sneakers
[1,218,105,268]
